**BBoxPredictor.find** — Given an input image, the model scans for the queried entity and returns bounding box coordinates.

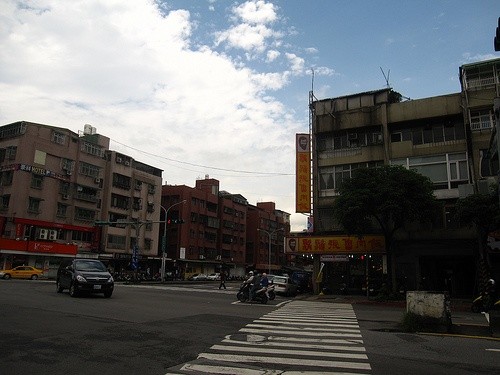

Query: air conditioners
[96,178,100,183]
[64,194,69,199]
[125,161,130,166]
[117,157,122,163]
[66,170,72,175]
[136,185,139,189]
[39,229,57,241]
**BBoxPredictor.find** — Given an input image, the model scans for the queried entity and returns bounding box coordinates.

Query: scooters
[268,285,275,300]
[471,290,500,313]
[237,281,246,299]
[239,283,269,305]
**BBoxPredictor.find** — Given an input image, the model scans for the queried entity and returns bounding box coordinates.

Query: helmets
[263,273,267,276]
[488,279,495,285]
[249,271,254,275]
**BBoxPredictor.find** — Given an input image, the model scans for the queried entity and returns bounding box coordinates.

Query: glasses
[249,273,252,274]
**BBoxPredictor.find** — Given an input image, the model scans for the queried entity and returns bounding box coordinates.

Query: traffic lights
[167,219,184,224]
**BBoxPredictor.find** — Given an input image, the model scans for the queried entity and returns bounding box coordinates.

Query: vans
[56,259,114,298]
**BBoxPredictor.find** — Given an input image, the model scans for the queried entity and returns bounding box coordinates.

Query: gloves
[243,281,245,283]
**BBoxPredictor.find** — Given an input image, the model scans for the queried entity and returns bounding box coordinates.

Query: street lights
[257,227,285,273]
[148,200,187,277]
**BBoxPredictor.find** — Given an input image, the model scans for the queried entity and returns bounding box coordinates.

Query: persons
[481,273,496,314]
[238,271,269,303]
[217,272,226,290]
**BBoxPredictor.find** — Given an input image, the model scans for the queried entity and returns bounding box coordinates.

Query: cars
[273,276,296,295]
[207,273,221,281]
[189,273,207,281]
[0,266,44,280]
